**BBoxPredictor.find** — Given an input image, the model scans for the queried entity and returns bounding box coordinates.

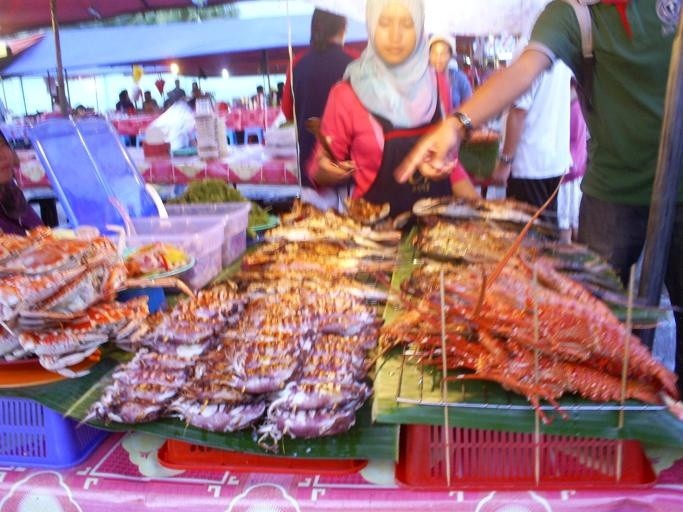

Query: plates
[120,247,193,278]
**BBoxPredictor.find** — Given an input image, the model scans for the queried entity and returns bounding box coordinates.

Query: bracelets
[498,153,512,164]
[450,109,473,146]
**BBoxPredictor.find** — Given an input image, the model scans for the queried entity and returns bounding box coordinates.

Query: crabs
[0,224,194,379]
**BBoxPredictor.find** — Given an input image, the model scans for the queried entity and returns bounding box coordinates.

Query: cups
[193,97,231,163]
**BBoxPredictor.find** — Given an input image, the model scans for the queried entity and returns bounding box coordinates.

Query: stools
[245,128,262,145]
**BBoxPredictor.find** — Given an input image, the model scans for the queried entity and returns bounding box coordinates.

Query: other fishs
[234,195,403,303]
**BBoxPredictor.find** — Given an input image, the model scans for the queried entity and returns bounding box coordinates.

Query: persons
[187,83,202,110]
[115,90,134,114]
[0,130,47,236]
[303,2,484,219]
[141,90,158,112]
[170,81,185,105]
[249,85,264,107]
[279,7,362,187]
[396,1,682,401]
[493,51,570,248]
[556,79,586,248]
[423,32,482,154]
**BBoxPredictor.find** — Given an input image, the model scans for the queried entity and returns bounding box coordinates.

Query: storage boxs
[27,117,251,291]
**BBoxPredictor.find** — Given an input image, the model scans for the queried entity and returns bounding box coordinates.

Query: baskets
[156,435,369,477]
[0,396,111,469]
[394,420,659,491]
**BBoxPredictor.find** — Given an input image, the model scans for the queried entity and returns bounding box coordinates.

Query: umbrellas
[154,72,165,98]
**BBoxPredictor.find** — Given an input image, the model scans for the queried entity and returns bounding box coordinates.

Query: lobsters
[367,169,681,426]
